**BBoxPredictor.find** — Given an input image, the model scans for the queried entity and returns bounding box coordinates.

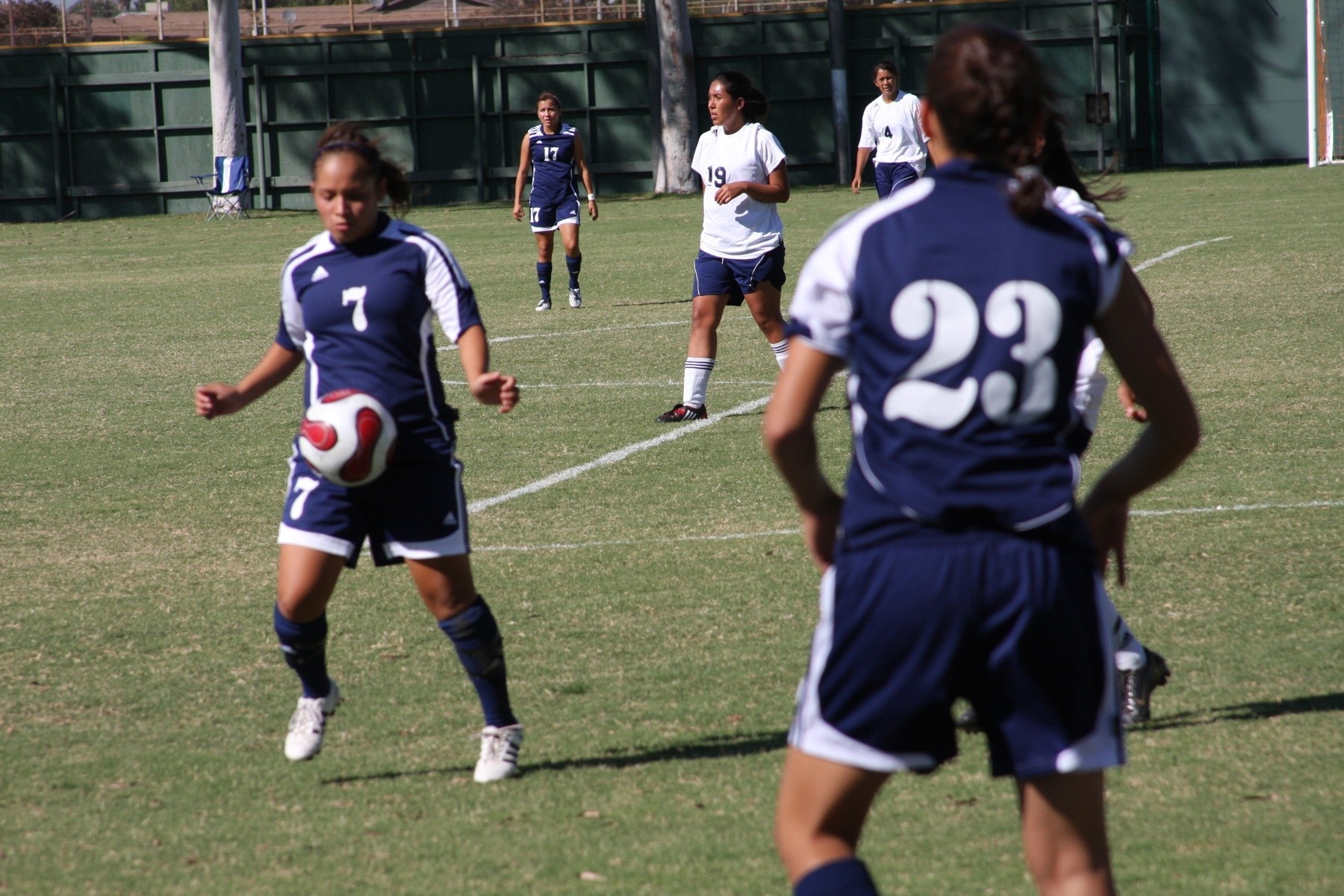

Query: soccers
[298,388,397,490]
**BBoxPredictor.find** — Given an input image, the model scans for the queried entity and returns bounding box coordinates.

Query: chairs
[190,156,250,221]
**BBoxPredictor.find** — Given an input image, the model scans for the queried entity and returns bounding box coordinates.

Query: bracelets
[587,193,595,201]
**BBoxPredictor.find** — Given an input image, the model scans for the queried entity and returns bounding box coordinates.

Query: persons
[510,92,598,313]
[655,71,789,424]
[194,123,525,784]
[761,29,1204,895]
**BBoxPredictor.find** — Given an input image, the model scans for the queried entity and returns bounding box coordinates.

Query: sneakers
[654,403,708,422]
[1117,646,1171,732]
[472,723,526,785]
[568,280,582,308]
[283,679,340,761]
[535,299,552,311]
